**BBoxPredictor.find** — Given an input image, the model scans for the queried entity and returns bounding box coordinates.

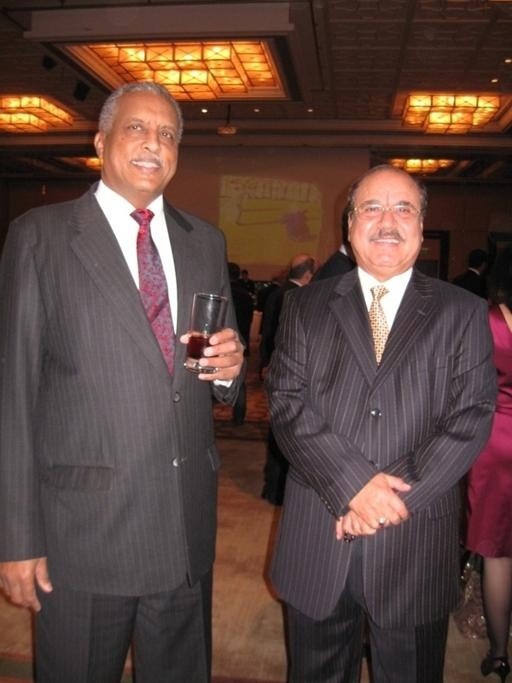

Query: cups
[183,293,229,375]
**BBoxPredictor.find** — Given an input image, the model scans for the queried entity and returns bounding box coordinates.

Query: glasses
[353,204,422,220]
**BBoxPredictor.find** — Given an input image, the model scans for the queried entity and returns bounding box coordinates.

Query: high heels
[480,650,512,683]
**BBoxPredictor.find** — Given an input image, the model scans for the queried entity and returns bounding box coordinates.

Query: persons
[222,206,355,508]
[1,81,247,682]
[451,246,512,683]
[261,164,499,682]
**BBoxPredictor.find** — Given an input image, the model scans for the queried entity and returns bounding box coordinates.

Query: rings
[377,516,386,526]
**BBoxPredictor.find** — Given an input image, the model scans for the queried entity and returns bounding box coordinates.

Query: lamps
[389,87,512,134]
[22,1,323,103]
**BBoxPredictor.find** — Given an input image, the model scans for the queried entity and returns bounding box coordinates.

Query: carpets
[209,347,271,442]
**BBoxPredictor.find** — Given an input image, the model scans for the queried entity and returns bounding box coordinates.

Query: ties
[367,286,390,368]
[128,207,177,380]
